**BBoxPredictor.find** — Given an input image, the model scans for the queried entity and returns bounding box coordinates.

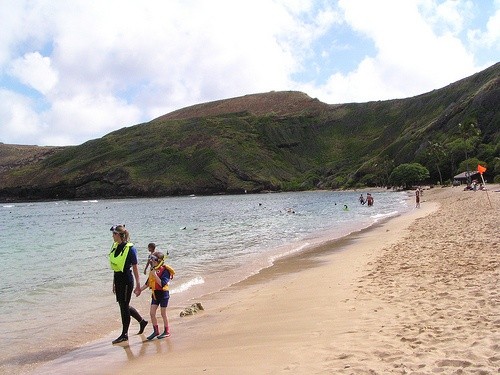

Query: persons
[135,252,171,339]
[415,187,423,208]
[465,183,484,190]
[358,193,374,206]
[108,224,148,344]
[144,243,156,275]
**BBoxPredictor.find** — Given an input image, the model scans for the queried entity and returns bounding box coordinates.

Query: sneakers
[112,332,128,344]
[147,331,159,340]
[158,330,170,339]
[139,319,148,334]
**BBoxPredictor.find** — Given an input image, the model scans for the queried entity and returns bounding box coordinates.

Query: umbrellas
[453,171,479,178]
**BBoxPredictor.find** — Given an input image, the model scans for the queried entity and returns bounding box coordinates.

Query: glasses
[110,224,123,234]
[151,257,163,261]
